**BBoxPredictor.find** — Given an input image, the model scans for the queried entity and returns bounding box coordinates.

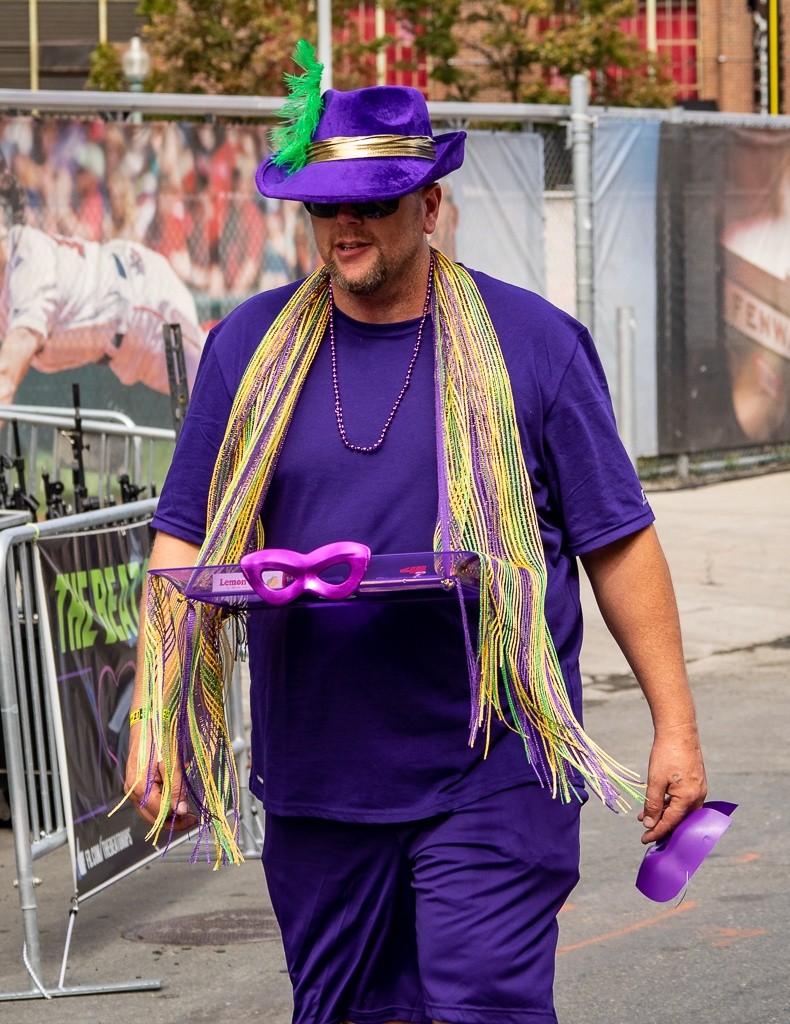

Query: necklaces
[329,258,434,453]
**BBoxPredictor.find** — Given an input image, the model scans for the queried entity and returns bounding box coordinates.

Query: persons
[122,85,740,1023]
[1,91,318,437]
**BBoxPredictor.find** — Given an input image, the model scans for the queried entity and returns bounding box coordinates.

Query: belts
[96,253,127,367]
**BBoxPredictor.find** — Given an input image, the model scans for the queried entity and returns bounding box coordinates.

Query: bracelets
[129,707,171,727]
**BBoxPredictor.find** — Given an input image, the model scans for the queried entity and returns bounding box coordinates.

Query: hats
[255,38,468,204]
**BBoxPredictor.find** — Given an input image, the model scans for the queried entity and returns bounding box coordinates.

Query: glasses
[303,197,400,218]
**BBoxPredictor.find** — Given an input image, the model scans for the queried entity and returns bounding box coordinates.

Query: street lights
[122,36,151,124]
